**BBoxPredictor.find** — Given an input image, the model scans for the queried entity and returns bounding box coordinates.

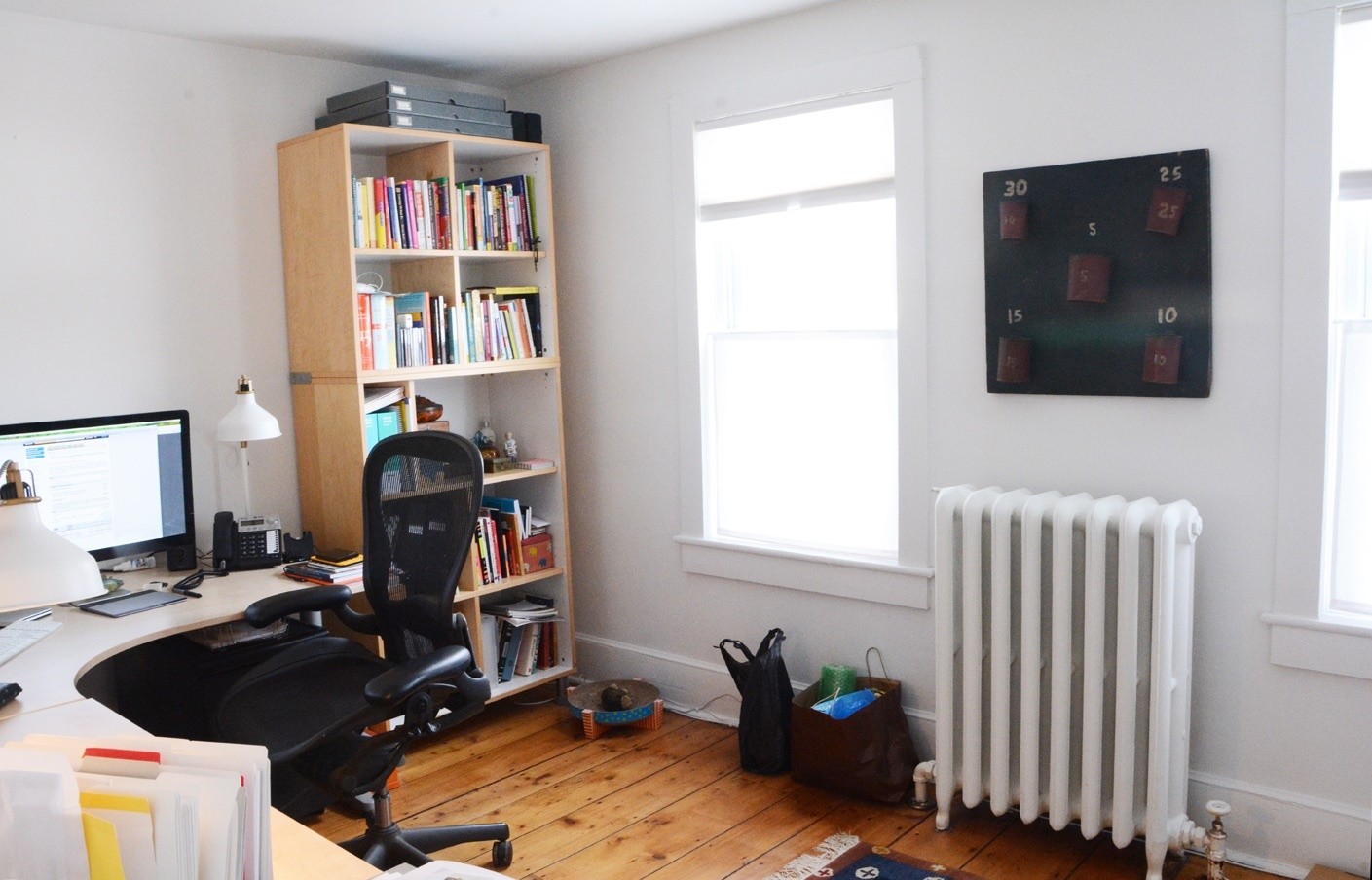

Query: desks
[0,559,385,880]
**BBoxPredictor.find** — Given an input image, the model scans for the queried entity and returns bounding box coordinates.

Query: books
[284,175,561,683]
[0,607,52,627]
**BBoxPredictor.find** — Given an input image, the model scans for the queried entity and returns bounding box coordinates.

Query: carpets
[765,832,992,880]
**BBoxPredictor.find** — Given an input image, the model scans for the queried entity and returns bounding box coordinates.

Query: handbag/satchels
[792,648,920,804]
[717,628,796,775]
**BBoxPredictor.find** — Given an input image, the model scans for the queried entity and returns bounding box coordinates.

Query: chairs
[206,430,513,872]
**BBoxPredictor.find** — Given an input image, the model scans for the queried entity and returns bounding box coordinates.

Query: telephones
[213,510,285,571]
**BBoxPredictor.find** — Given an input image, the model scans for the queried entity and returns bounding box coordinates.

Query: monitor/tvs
[0,410,198,561]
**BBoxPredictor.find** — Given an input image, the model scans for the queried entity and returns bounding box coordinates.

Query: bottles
[113,556,156,572]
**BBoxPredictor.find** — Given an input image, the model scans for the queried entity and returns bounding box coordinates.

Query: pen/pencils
[172,587,202,598]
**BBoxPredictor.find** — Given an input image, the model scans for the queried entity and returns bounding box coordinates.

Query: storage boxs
[520,533,555,575]
[315,80,514,140]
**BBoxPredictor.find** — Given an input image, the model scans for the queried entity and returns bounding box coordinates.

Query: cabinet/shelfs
[277,122,578,740]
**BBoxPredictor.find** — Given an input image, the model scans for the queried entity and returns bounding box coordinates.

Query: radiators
[935,483,1201,880]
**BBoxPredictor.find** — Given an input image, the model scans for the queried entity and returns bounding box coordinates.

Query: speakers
[166,542,197,570]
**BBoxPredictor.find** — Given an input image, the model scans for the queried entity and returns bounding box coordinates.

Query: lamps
[213,374,282,516]
[0,459,109,615]
